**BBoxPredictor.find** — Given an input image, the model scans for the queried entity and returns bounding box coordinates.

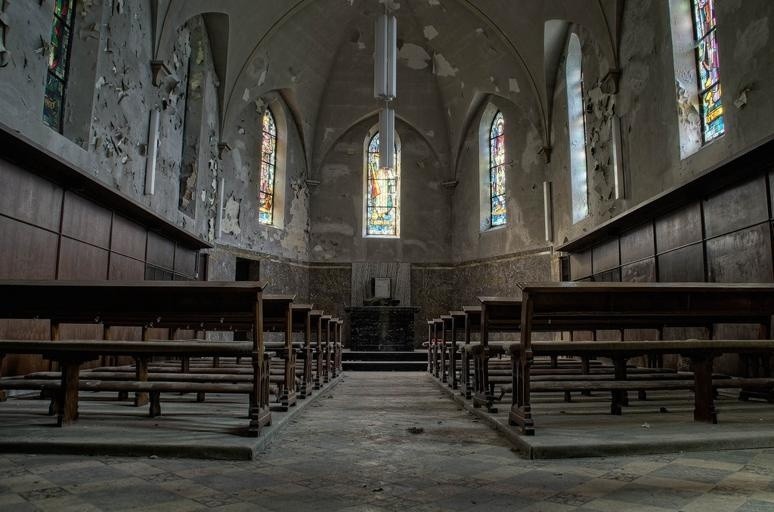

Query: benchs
[422,282,774,437]
[0,279,343,437]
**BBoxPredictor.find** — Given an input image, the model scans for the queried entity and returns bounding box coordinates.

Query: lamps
[542,181,553,243]
[144,110,159,197]
[214,177,223,239]
[372,1,399,170]
[609,116,625,201]
